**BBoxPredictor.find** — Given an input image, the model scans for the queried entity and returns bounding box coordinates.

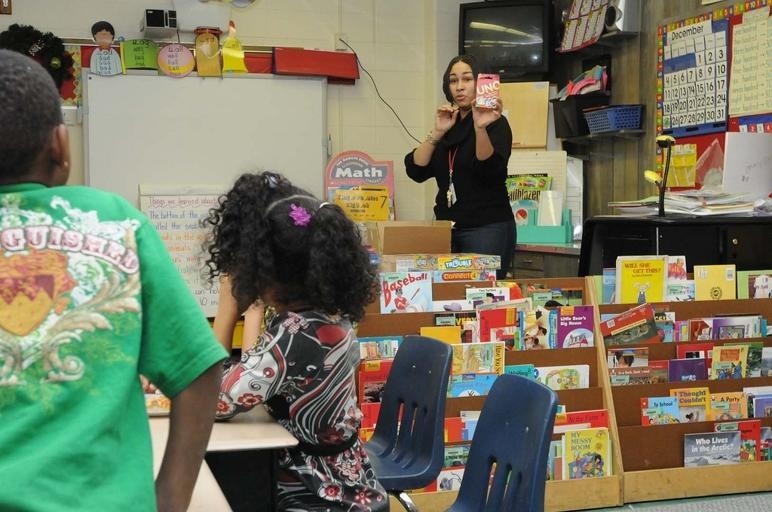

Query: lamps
[644,135,676,217]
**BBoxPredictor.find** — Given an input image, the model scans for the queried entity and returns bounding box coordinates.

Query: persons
[0,48,230,512]
[198,170,390,512]
[404,55,517,280]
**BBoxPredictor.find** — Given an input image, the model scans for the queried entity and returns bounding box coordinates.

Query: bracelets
[424,135,440,146]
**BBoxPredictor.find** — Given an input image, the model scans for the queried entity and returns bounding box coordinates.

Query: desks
[145,390,299,512]
[578,216,772,278]
[506,240,582,278]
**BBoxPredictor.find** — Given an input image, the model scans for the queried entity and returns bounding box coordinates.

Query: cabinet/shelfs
[555,30,646,147]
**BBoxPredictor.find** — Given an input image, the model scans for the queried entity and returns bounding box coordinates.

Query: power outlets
[335,32,348,50]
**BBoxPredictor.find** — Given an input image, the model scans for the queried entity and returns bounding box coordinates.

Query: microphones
[450,102,460,114]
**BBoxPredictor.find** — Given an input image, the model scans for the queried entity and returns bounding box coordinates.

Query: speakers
[167,9,177,28]
[145,9,164,27]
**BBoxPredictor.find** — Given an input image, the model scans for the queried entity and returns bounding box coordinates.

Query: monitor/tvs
[457,0,554,82]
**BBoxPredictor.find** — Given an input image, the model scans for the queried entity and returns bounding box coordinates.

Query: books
[607,189,772,220]
[360,253,613,491]
[594,255,771,465]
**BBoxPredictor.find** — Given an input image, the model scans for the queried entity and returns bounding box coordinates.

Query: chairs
[444,373,559,512]
[363,335,453,512]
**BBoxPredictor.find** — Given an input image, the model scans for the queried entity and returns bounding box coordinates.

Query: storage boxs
[549,89,609,138]
[366,222,452,273]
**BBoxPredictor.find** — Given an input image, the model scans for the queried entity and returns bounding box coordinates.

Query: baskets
[584,106,642,135]
